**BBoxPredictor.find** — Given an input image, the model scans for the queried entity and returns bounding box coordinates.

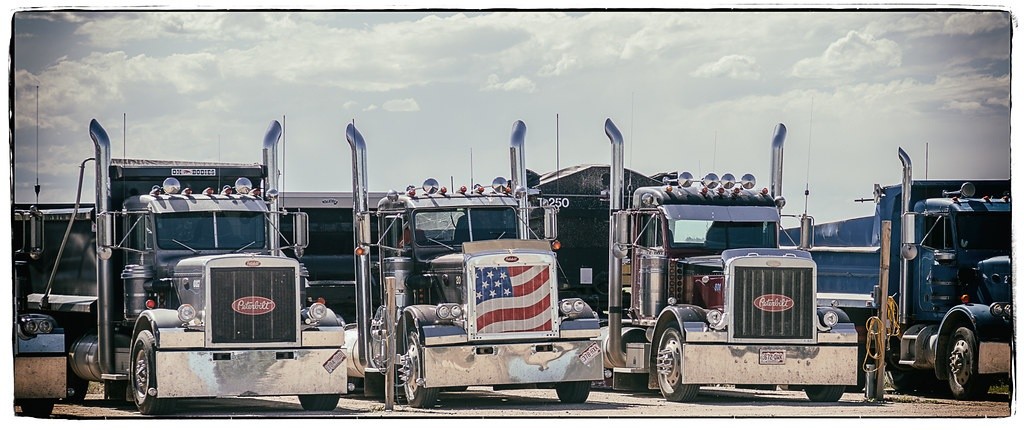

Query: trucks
[780,146,1012,403]
[12,117,347,415]
[278,120,605,408]
[533,117,859,406]
